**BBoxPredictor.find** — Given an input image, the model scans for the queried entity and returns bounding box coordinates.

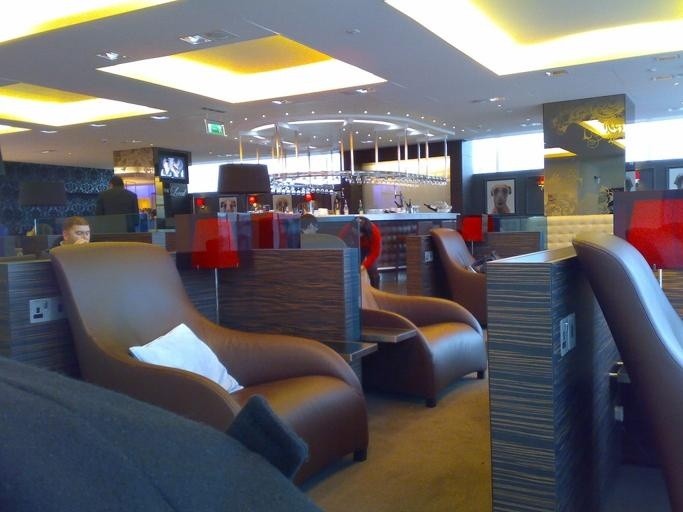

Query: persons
[343,199,349,214]
[160,158,184,178]
[300,214,320,234]
[336,198,341,215]
[357,200,364,214]
[57,216,91,247]
[94,178,140,232]
[339,216,381,291]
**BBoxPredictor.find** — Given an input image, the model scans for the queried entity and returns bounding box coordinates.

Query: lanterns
[196,199,205,206]
[305,194,314,204]
[248,197,257,207]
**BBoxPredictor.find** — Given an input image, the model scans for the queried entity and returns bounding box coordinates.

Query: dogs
[490,184,511,213]
[221,199,236,213]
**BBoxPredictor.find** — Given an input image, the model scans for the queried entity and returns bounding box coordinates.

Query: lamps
[459,215,483,250]
[623,196,682,289]
[189,219,240,313]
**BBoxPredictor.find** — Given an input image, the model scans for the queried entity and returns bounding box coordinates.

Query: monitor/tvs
[158,150,189,183]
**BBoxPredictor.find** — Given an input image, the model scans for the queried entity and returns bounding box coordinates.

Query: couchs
[51,242,368,489]
[430,229,487,326]
[361,268,486,407]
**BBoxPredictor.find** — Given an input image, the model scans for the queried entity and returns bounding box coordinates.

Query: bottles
[423,203,439,212]
[332,197,365,216]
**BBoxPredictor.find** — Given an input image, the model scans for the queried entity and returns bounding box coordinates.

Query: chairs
[571,229,682,512]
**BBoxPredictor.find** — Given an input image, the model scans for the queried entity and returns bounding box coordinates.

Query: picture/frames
[483,177,517,217]
